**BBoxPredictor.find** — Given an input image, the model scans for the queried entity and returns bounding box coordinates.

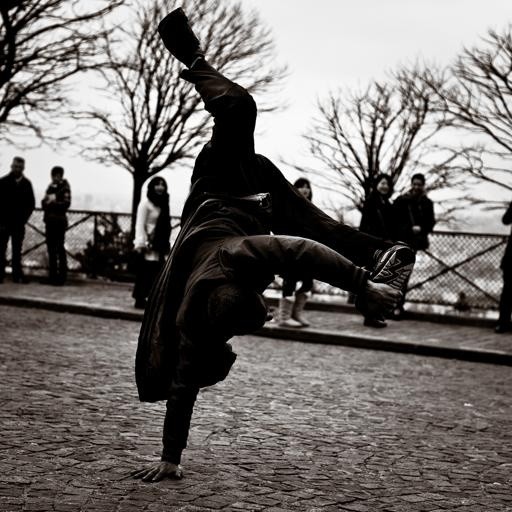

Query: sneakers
[354,240,415,321]
[158,8,201,62]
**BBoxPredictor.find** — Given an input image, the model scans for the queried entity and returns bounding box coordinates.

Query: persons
[129,172,175,308]
[392,174,435,319]
[355,169,393,326]
[494,198,512,333]
[276,174,317,331]
[1,157,35,285]
[39,163,73,287]
[124,4,414,484]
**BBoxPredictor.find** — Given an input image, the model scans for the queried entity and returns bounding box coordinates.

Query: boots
[278,295,302,328]
[292,291,312,327]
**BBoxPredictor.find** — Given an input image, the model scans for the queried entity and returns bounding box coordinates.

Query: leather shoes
[494,322,505,333]
[12,271,31,283]
[363,318,387,328]
[54,273,68,285]
[134,298,148,309]
